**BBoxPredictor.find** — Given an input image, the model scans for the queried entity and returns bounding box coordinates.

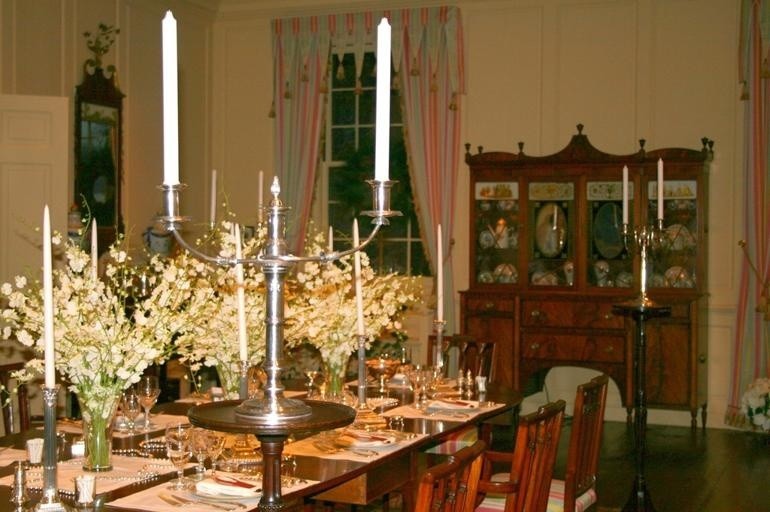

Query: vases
[215,364,248,400]
[75,390,123,472]
[321,351,349,400]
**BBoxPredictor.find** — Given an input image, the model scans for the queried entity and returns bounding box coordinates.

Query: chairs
[414,440,486,511]
[0,362,30,435]
[478,399,565,512]
[476,373,610,512]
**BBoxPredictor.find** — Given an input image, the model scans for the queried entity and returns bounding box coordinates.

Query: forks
[157,494,236,512]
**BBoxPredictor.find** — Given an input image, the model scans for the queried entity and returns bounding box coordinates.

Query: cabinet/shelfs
[464,125,715,291]
[518,296,626,390]
[461,296,516,388]
[626,296,692,407]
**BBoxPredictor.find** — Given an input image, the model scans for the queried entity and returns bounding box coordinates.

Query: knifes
[172,494,247,509]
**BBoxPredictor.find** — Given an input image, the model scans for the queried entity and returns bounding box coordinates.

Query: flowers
[1,195,226,390]
[175,255,319,369]
[277,236,410,354]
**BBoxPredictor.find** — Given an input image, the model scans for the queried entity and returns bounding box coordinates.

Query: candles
[622,165,630,226]
[258,171,264,222]
[376,18,392,182]
[436,224,443,321]
[352,219,365,335]
[161,11,180,185]
[655,157,665,223]
[327,225,334,257]
[210,168,217,221]
[232,224,247,362]
[42,203,57,387]
[91,217,99,279]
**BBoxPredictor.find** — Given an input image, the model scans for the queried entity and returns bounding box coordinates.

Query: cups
[122,395,140,436]
[137,376,159,433]
[26,438,44,463]
[165,421,195,491]
[75,477,97,507]
[206,439,227,478]
[137,388,161,427]
[190,433,208,482]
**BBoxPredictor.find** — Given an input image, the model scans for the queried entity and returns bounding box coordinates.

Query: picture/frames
[74,61,126,233]
